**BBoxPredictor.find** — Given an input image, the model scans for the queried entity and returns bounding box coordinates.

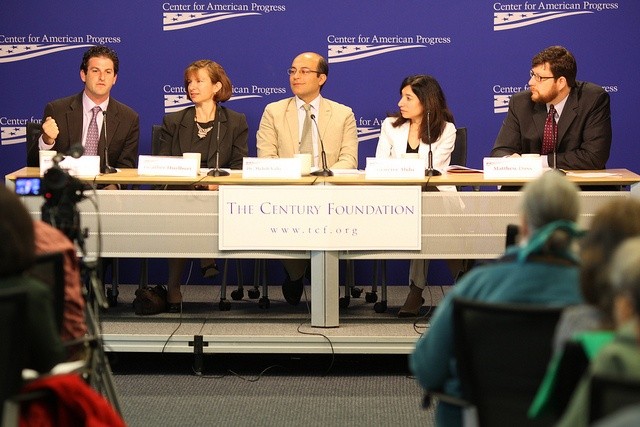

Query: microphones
[546,104,566,174]
[100,110,117,173]
[310,113,333,176]
[424,109,441,175]
[208,105,229,176]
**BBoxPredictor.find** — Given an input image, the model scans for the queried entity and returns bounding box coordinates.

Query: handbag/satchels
[132,283,166,315]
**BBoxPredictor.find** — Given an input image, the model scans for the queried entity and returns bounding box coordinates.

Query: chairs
[137,119,244,312]
[21,120,120,311]
[247,257,364,313]
[421,293,581,425]
[366,125,467,314]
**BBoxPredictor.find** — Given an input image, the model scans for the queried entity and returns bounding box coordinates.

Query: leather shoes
[202,261,219,278]
[398,295,425,316]
[167,292,182,313]
[282,275,304,306]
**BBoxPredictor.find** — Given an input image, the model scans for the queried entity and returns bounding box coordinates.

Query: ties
[85,107,102,156]
[300,104,314,168]
[542,105,558,155]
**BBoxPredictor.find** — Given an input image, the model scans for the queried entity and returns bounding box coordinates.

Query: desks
[0,166,639,328]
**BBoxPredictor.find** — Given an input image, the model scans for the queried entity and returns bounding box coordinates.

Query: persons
[156,59,249,313]
[374,74,457,317]
[410,169,584,426]
[0,184,127,427]
[552,237,640,426]
[256,52,359,304]
[494,45,611,191]
[552,197,640,426]
[26,45,140,190]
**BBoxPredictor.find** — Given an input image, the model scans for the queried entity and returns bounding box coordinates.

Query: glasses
[287,66,325,75]
[529,70,557,82]
